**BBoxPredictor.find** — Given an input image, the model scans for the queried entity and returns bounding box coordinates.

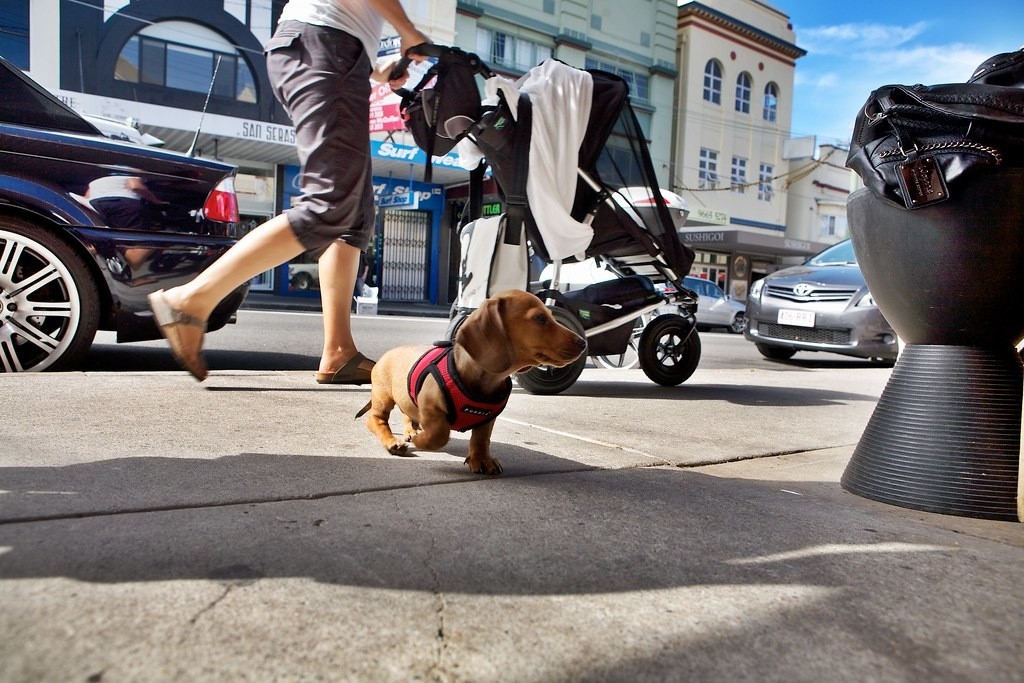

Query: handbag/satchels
[455,92,532,314]
[844,44,1024,211]
[399,45,482,158]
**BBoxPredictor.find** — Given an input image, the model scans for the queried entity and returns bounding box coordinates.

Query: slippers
[147,288,210,380]
[316,350,376,385]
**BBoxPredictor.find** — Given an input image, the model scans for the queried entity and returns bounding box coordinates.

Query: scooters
[451,185,690,369]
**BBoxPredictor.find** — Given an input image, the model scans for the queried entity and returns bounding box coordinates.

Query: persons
[85,172,170,279]
[147,0,433,384]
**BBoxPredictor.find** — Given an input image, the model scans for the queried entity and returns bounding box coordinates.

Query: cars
[747,232,900,364]
[652,277,748,335]
[0,56,253,373]
[287,252,321,290]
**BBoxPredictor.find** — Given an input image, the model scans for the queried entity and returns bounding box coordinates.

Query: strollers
[389,42,703,395]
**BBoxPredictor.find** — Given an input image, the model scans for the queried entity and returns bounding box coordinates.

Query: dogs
[352,290,587,475]
